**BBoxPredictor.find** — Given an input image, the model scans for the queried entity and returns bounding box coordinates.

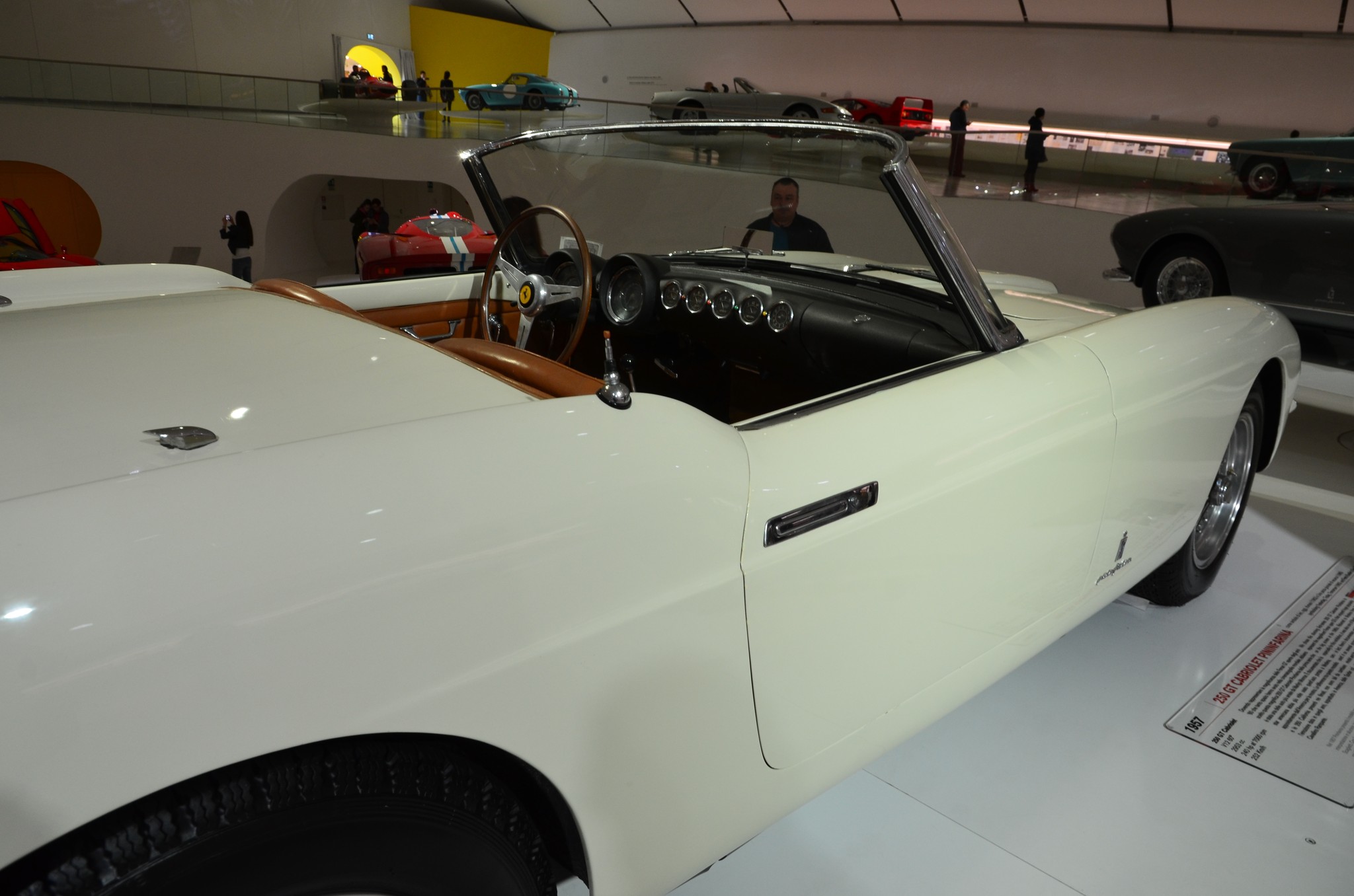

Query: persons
[350,65,392,84]
[949,100,972,177]
[1023,108,1047,192]
[219,209,254,284]
[742,177,836,254]
[349,198,390,275]
[703,81,714,92]
[440,71,454,123]
[416,71,429,124]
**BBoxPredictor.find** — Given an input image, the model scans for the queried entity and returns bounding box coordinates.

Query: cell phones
[225,214,231,220]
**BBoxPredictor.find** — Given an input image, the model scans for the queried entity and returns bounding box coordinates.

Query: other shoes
[442,118,446,122]
[448,119,450,123]
[949,172,965,177]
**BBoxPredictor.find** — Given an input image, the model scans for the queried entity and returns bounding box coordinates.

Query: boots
[1024,181,1038,192]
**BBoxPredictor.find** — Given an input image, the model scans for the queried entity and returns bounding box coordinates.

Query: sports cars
[830,96,934,141]
[1104,203,1353,370]
[0,197,106,272]
[353,69,398,100]
[650,77,854,138]
[1227,128,1354,201]
[0,119,1302,896]
[459,72,580,111]
[356,212,548,280]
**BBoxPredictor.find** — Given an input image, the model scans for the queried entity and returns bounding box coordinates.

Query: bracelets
[223,224,227,228]
